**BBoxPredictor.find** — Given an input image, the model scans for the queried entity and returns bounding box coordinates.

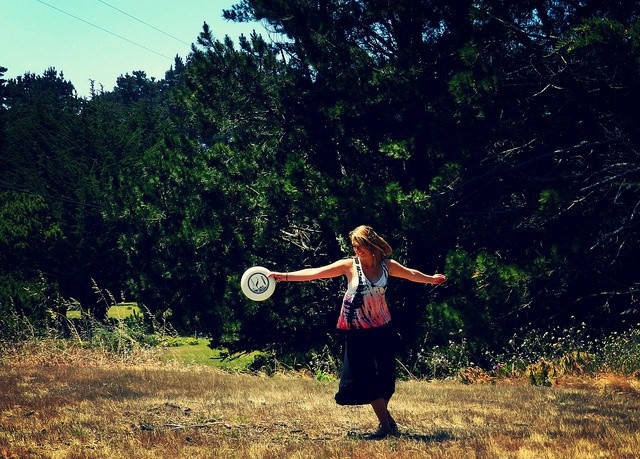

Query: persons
[266,226,447,439]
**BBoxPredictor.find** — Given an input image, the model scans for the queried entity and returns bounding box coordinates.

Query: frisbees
[240,266,275,301]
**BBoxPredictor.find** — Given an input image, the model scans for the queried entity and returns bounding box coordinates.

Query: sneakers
[363,419,398,439]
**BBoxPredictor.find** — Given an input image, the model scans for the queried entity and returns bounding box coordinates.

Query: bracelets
[286,273,288,282]
